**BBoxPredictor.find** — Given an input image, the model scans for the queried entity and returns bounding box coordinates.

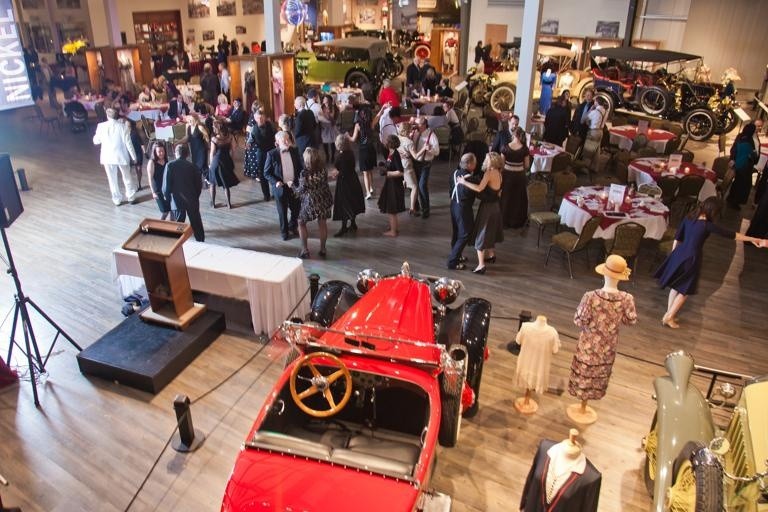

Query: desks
[114,241,303,339]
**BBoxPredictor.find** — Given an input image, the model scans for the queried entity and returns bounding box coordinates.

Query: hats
[595,255,632,281]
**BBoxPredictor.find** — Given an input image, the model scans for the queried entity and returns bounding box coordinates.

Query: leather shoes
[455,263,466,270]
[459,255,468,263]
[282,232,289,239]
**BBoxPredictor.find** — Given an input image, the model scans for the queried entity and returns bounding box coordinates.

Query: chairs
[253,419,350,466]
[333,432,420,477]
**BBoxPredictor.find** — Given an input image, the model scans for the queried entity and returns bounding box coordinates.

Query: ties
[415,133,421,153]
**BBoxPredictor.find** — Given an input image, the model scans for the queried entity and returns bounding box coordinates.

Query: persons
[567,251,639,422]
[405,55,459,127]
[244,79,438,258]
[519,426,601,511]
[26,39,265,241]
[655,196,763,330]
[445,89,759,273]
[514,315,560,414]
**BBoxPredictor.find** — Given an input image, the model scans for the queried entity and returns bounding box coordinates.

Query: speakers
[0,151,25,230]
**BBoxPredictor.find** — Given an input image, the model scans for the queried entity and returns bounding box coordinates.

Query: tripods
[0,231,86,406]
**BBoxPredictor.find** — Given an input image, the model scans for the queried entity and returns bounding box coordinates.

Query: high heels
[471,265,487,275]
[347,224,357,233]
[334,227,348,237]
[296,247,310,258]
[409,209,420,217]
[318,251,326,258]
[663,317,679,329]
[484,255,496,264]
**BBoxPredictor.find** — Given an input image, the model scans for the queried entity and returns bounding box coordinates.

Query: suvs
[590,46,739,141]
[218,262,493,512]
[291,38,391,90]
[469,43,593,112]
[411,38,432,61]
[638,345,766,512]
